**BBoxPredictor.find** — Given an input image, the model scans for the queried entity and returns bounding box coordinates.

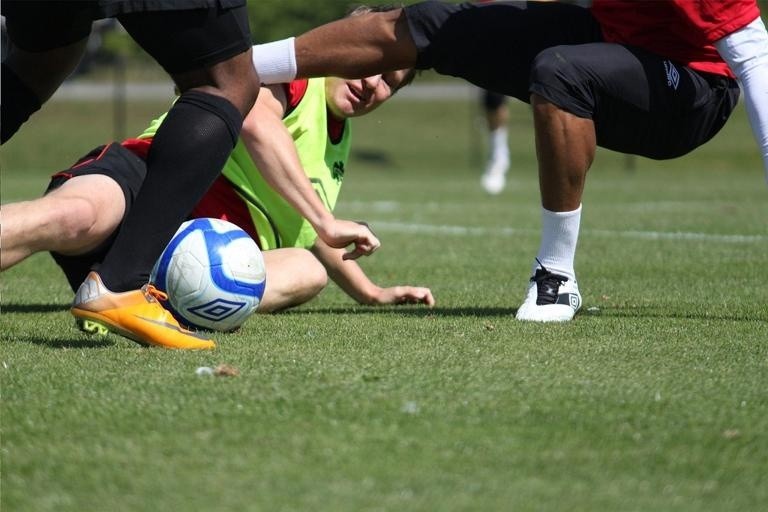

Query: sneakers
[515,254,581,323]
[69,270,216,351]
[480,127,511,194]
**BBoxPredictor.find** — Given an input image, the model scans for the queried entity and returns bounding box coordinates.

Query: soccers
[151,216,266,332]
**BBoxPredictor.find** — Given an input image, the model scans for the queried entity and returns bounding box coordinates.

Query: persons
[0,0,260,350]
[0,0,435,335]
[477,84,511,195]
[173,0,767,325]
